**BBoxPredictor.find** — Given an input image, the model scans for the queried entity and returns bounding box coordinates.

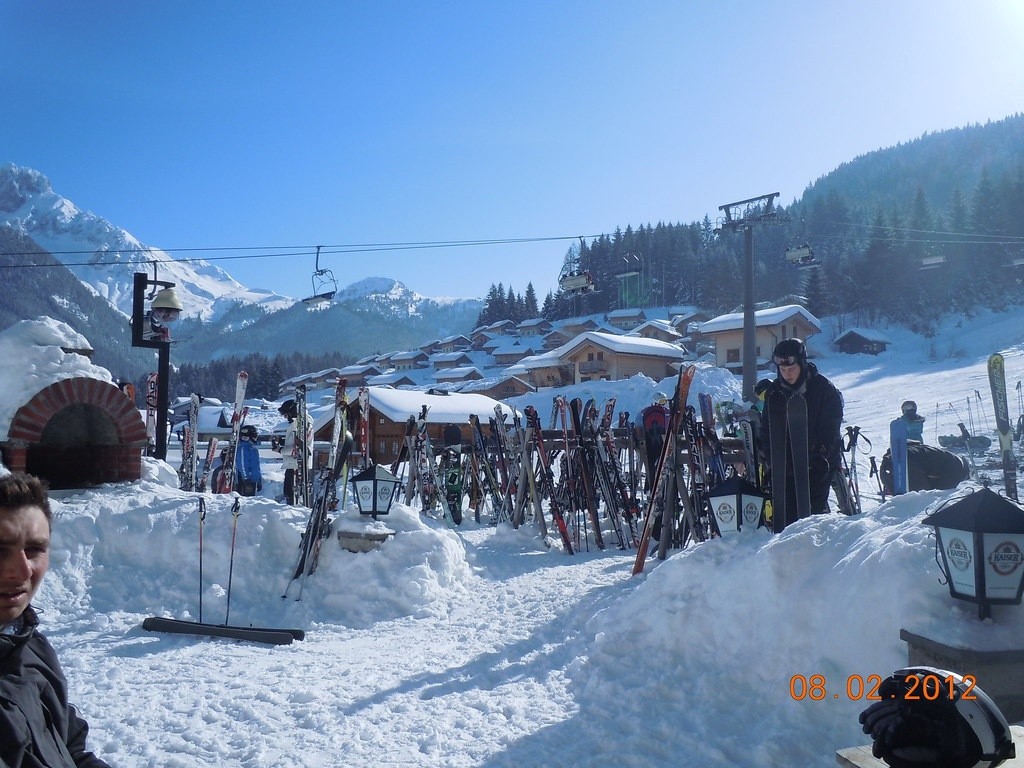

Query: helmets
[901,401,917,411]
[892,666,1016,768]
[654,391,668,406]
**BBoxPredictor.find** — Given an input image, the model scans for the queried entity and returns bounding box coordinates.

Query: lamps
[921,480,1024,620]
[705,474,766,537]
[348,464,402,520]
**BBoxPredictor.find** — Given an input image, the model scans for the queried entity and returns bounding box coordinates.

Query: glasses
[771,354,796,366]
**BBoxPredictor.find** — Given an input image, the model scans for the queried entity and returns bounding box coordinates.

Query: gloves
[257,483,262,492]
[860,698,979,768]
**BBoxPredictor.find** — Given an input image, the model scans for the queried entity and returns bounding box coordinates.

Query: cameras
[272,436,277,450]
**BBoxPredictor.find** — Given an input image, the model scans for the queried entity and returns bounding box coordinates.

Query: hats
[773,338,808,373]
[277,398,297,423]
[240,425,257,441]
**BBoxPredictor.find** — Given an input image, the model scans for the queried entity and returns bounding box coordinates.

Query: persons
[210,399,314,505]
[0,471,110,768]
[635,338,925,533]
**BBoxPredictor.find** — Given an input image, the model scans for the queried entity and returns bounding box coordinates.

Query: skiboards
[986,352,1019,500]
[115,360,992,585]
[142,615,306,646]
